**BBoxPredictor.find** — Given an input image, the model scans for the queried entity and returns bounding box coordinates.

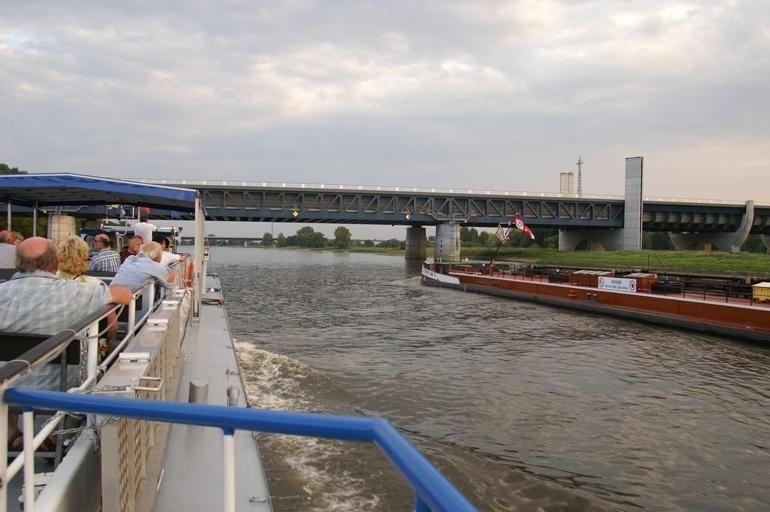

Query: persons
[153,236,166,251]
[155,237,185,300]
[57,234,118,364]
[88,234,121,272]
[1,237,132,463]
[119,235,144,265]
[110,242,175,336]
[1,230,25,268]
[131,216,156,244]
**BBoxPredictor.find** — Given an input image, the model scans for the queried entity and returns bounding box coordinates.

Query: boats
[420,259,770,343]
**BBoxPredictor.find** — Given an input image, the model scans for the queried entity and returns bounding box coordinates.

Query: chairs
[1,236,174,470]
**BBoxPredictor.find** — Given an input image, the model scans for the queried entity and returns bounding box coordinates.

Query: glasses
[94,239,101,242]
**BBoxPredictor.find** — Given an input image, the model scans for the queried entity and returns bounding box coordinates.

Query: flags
[511,211,535,240]
